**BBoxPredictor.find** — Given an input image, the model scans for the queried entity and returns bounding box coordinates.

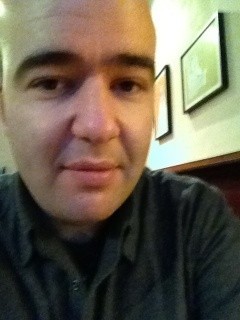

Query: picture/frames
[153,65,173,141]
[180,10,229,115]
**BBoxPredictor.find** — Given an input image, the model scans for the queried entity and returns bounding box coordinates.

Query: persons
[0,0,240,320]
